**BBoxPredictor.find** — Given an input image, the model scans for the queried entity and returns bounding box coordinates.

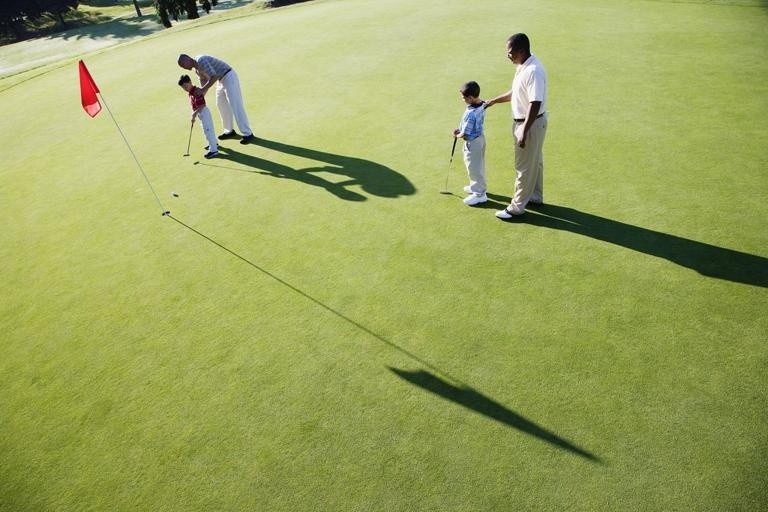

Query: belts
[218,68,232,81]
[513,111,545,122]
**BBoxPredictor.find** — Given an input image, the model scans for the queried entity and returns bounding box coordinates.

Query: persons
[176,53,254,145]
[452,81,489,206]
[484,31,549,222]
[177,74,220,158]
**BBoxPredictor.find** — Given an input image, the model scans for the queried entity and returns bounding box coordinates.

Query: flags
[78,60,102,119]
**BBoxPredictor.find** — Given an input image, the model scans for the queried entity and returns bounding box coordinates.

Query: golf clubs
[440,137,458,194]
[183,117,195,155]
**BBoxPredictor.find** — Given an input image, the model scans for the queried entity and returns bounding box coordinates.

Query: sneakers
[495,208,528,220]
[463,184,488,206]
[204,143,219,159]
[218,130,237,140]
[240,133,254,144]
